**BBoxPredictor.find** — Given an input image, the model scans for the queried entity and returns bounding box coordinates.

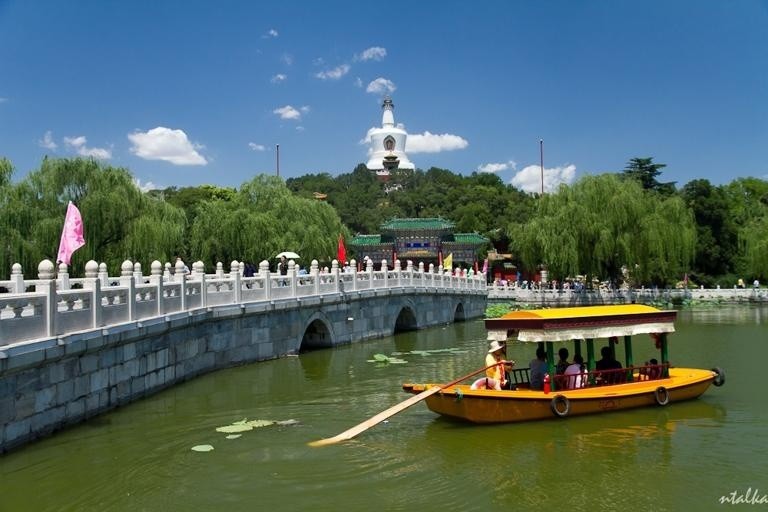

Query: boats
[402,303,725,424]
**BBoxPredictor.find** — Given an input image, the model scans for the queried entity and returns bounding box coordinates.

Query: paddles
[338,360,513,440]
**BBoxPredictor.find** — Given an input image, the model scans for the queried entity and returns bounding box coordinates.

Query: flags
[481,258,488,273]
[336,235,346,266]
[443,253,453,270]
[54,200,86,266]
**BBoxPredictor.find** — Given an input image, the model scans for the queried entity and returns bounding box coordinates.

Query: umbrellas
[274,251,301,260]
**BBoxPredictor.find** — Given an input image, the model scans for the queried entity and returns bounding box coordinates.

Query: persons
[753,277,761,288]
[492,276,622,293]
[484,340,520,391]
[174,255,191,276]
[528,346,548,390]
[737,276,744,288]
[554,347,572,389]
[645,358,661,378]
[595,346,624,384]
[562,352,589,388]
[432,265,475,289]
[241,255,351,289]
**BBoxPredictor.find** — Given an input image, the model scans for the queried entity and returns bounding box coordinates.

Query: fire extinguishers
[543,374,549,394]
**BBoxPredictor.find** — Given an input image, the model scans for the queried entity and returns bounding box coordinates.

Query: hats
[488,341,505,353]
